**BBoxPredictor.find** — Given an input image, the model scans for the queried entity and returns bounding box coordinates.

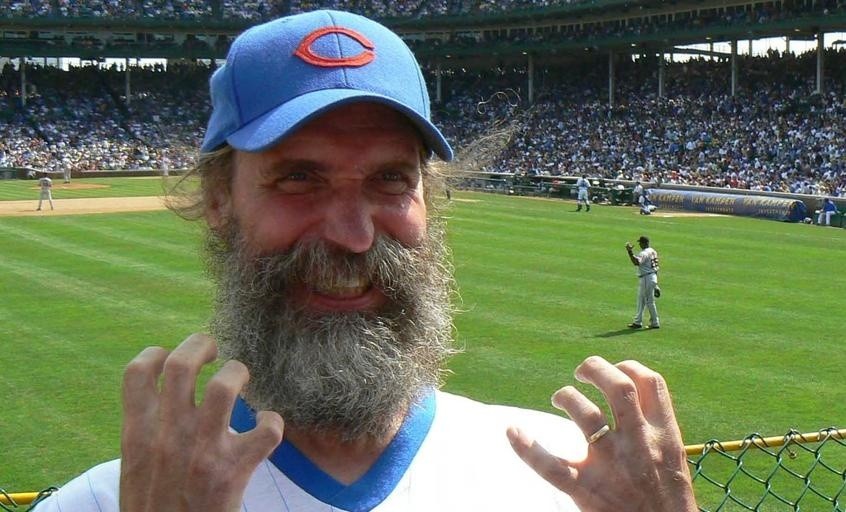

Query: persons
[625,234,662,330]
[157,155,170,177]
[1,0,308,173]
[817,198,839,227]
[31,6,701,511]
[34,174,53,211]
[60,154,79,183]
[638,189,657,215]
[575,174,593,212]
[303,0,846,198]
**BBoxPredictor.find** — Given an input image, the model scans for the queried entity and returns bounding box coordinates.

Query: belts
[638,273,651,278]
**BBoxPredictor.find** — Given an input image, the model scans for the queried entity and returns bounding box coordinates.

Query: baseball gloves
[655,286,661,298]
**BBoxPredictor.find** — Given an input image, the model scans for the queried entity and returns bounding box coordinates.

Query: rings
[586,424,610,445]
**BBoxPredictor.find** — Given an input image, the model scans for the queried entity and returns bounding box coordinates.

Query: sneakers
[627,323,659,328]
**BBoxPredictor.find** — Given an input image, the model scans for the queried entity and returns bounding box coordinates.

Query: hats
[637,236,650,242]
[201,10,454,164]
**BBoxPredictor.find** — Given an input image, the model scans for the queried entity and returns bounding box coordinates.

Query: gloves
[625,242,635,250]
[654,287,661,298]
